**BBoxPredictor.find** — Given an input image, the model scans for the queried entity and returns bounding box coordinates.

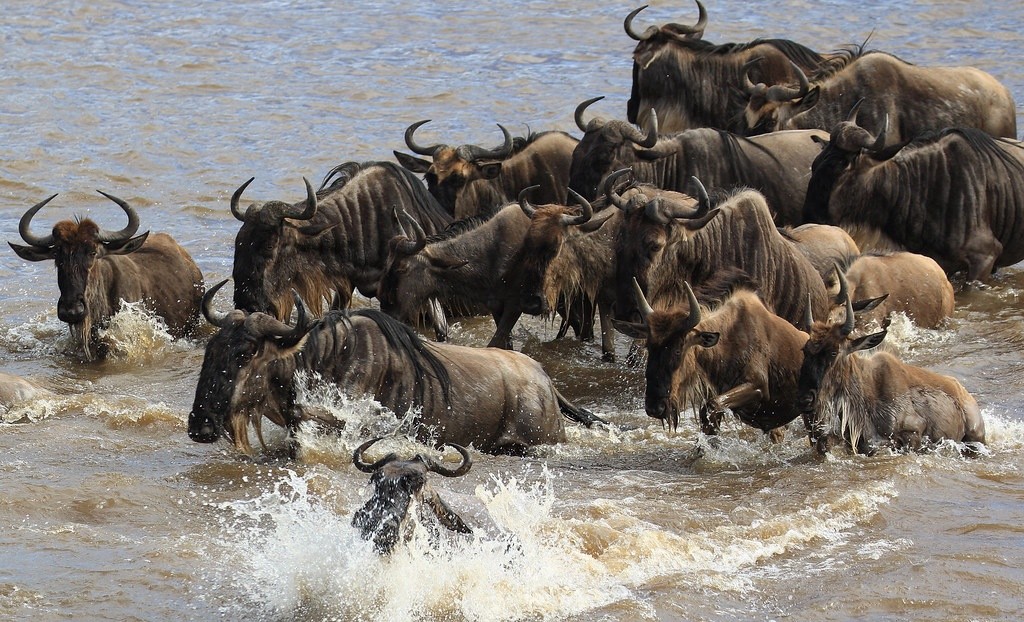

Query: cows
[7,190,205,360]
[188,2,1024,551]
